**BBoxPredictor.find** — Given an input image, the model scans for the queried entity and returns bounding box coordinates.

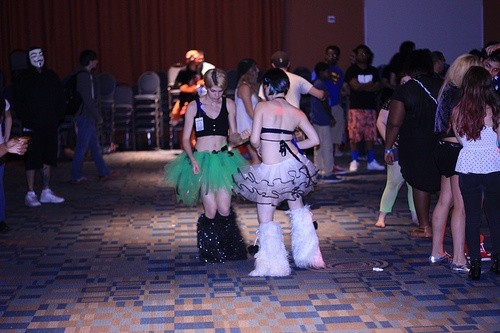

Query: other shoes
[25,192,41,207]
[408,226,432,238]
[68,178,88,184]
[40,189,64,203]
[350,160,360,173]
[316,174,343,182]
[99,171,120,181]
[366,160,385,170]
[464,243,470,260]
[452,260,472,273]
[333,166,346,174]
[429,251,453,266]
[481,243,492,261]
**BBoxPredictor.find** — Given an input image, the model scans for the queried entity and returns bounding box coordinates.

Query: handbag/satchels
[61,69,89,117]
[421,133,462,177]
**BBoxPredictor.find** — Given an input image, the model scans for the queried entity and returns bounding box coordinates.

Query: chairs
[56,60,216,161]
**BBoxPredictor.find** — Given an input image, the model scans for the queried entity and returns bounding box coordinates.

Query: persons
[257,52,328,157]
[20,45,67,208]
[172,50,207,151]
[245,68,326,276]
[312,40,500,283]
[0,90,29,233]
[235,57,263,166]
[182,68,247,261]
[72,48,121,185]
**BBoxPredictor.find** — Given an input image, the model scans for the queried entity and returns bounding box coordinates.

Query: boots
[196,213,228,264]
[289,205,326,268]
[214,210,247,259]
[248,221,292,277]
